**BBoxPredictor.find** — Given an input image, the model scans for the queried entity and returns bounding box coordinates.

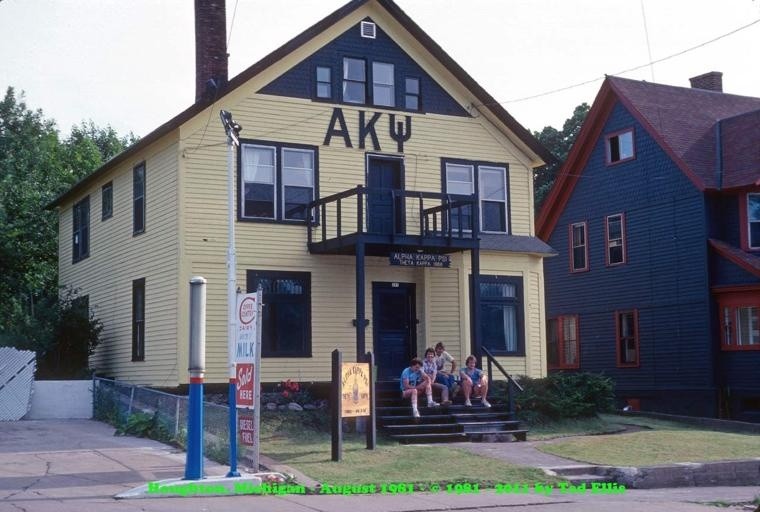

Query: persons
[399,356,441,418]
[459,354,492,408]
[432,341,457,396]
[422,346,453,405]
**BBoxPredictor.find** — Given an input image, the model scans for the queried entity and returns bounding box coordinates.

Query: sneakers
[427,402,441,408]
[412,410,421,418]
[464,399,473,407]
[441,400,453,406]
[480,400,492,409]
